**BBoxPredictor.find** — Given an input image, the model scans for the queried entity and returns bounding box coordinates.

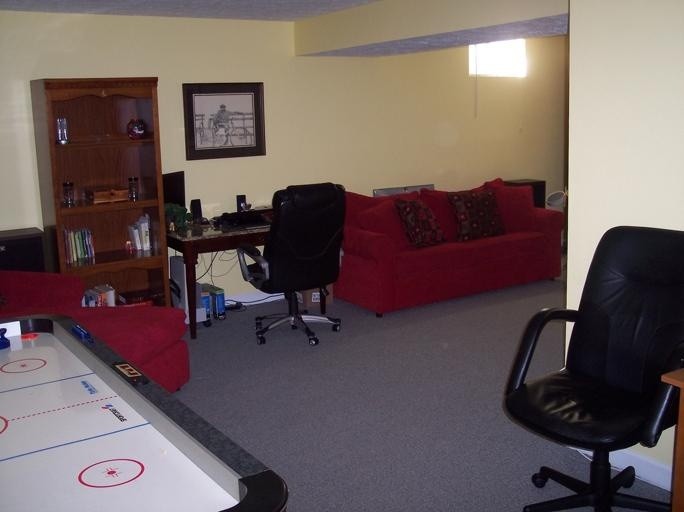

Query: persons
[215,104,231,135]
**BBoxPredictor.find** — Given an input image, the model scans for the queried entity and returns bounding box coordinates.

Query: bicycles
[210,118,252,147]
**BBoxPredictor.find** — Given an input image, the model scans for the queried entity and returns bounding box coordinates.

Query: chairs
[235,181,346,345]
[0,268,191,393]
[503,225,684,512]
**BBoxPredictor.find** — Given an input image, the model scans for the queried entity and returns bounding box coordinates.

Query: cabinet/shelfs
[29,76,171,306]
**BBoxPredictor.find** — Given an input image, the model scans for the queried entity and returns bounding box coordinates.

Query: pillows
[356,179,533,249]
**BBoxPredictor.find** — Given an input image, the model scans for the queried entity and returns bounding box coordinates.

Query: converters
[235,302,241,309]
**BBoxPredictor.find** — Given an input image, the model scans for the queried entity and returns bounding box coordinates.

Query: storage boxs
[295,283,333,307]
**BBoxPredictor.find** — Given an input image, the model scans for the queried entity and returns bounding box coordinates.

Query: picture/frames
[181,82,266,161]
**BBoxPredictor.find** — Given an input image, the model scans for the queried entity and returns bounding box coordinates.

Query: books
[128,218,152,250]
[81,285,163,306]
[63,225,95,264]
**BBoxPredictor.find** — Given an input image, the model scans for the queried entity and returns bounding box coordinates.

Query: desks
[0,228,46,266]
[504,179,546,210]
[166,218,326,339]
[0,312,290,512]
[661,369,684,512]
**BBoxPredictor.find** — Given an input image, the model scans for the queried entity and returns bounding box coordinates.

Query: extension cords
[225,304,242,311]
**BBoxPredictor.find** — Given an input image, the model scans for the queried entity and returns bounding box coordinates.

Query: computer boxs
[170,255,207,324]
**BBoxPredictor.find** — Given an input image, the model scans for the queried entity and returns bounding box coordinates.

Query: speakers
[191,199,202,220]
[237,194,246,211]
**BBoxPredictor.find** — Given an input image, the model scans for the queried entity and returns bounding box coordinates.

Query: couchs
[334,206,565,318]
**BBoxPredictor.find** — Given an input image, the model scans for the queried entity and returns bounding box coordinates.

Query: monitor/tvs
[162,170,185,207]
